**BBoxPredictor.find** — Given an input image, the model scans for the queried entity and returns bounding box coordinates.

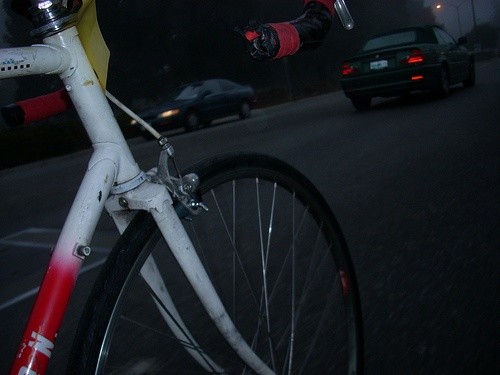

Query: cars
[133,78,260,139]
[340,27,478,108]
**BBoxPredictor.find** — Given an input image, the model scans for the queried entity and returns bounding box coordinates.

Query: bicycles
[0,0,365,375]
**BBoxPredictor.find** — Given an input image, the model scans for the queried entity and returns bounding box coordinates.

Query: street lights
[435,1,468,40]
